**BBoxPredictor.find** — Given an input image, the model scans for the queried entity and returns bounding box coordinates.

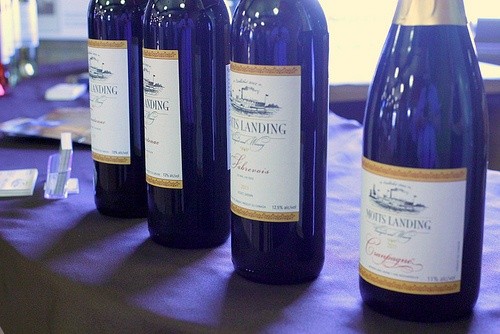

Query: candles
[0,0,39,65]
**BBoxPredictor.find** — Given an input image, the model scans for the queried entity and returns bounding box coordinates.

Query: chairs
[474,18,500,65]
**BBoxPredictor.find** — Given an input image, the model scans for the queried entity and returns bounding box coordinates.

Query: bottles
[87,0,148,219]
[0,0,39,88]
[357,0,488,322]
[137,0,230,249]
[231,0,329,284]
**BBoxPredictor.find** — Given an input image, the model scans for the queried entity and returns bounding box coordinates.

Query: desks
[0,60,500,334]
